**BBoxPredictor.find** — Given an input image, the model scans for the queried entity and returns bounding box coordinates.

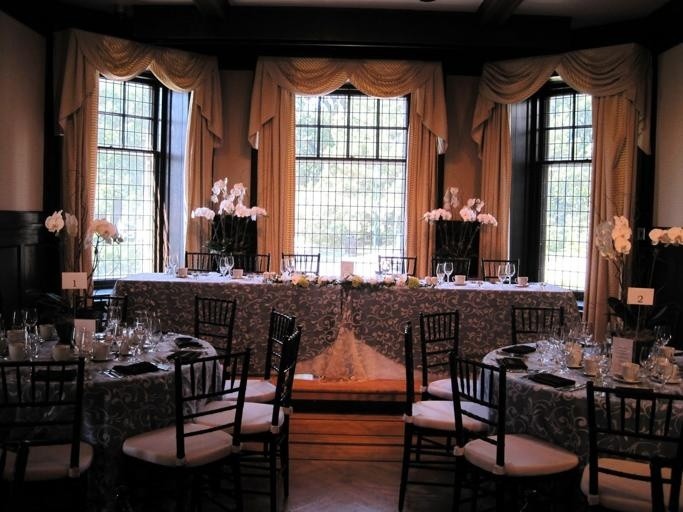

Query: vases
[213,250,247,272]
[433,258,471,281]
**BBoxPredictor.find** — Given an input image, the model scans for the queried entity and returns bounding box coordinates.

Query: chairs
[230,252,271,274]
[378,255,417,276]
[185,250,219,273]
[282,252,320,274]
[482,256,521,285]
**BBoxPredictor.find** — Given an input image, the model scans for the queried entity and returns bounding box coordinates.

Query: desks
[110,273,582,380]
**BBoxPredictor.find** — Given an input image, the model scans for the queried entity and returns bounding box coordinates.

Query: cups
[26,333,41,358]
[232,269,244,278]
[515,277,529,285]
[452,274,466,284]
[40,324,53,337]
[51,345,71,361]
[8,342,25,359]
[178,268,189,277]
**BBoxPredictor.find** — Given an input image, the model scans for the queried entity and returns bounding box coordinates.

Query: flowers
[290,274,419,291]
[190,177,268,250]
[422,187,498,257]
[26,209,124,315]
[595,215,683,333]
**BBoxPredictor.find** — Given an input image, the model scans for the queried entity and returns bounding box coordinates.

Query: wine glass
[436,262,453,287]
[72,305,162,362]
[219,256,235,279]
[382,260,391,276]
[13,309,38,331]
[164,256,178,278]
[279,257,294,277]
[497,264,515,288]
[536,320,674,392]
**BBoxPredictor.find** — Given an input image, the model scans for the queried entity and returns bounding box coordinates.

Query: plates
[516,285,530,288]
[454,284,467,286]
